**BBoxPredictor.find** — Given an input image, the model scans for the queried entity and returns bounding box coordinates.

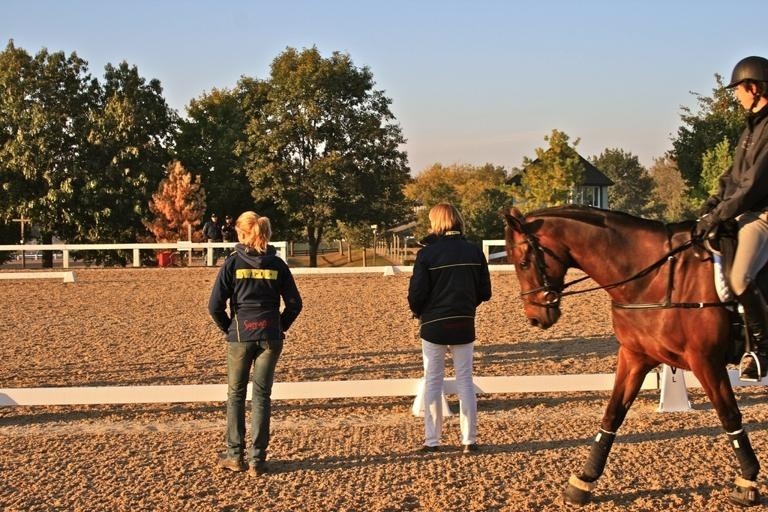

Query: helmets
[723,56,767,89]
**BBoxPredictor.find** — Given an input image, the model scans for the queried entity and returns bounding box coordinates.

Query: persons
[201,212,223,267]
[403,199,494,459]
[206,209,306,480]
[221,215,238,258]
[694,55,768,382]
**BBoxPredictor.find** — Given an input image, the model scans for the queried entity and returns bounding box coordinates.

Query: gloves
[693,207,706,219]
[695,212,721,241]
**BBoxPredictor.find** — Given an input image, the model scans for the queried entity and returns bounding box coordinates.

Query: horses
[498,202,768,509]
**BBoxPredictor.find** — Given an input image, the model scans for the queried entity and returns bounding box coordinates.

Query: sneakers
[218,458,247,472]
[415,446,440,455]
[249,461,269,477]
[463,442,477,453]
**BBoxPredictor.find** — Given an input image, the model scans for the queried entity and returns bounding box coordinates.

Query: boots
[733,279,767,379]
